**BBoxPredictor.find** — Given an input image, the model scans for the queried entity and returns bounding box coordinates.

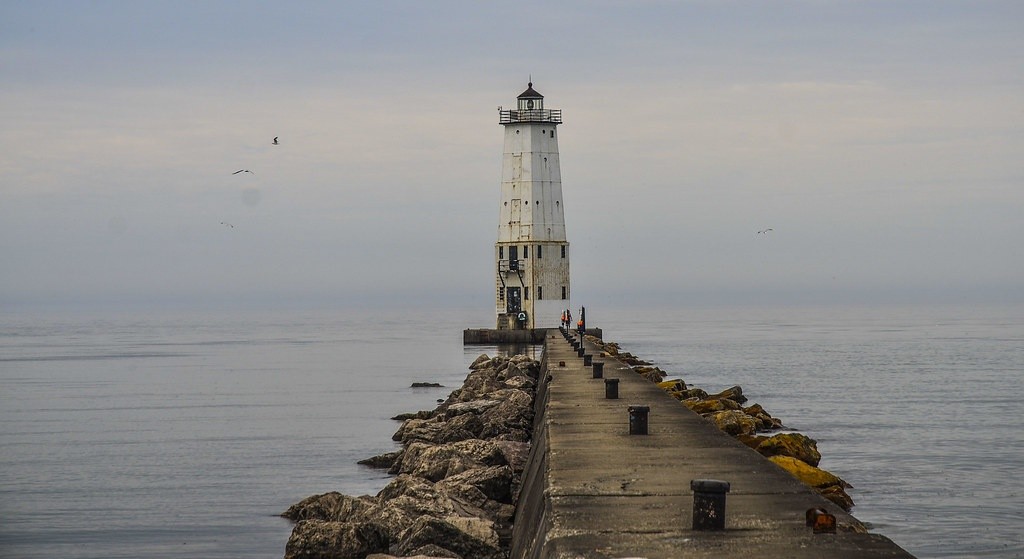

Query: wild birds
[272,136,281,145]
[232,169,256,176]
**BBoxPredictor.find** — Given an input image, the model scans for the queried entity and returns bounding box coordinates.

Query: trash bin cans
[593,362,604,378]
[584,355,593,366]
[578,348,585,357]
[628,405,650,435]
[559,326,580,351]
[691,478,730,531]
[604,379,620,399]
